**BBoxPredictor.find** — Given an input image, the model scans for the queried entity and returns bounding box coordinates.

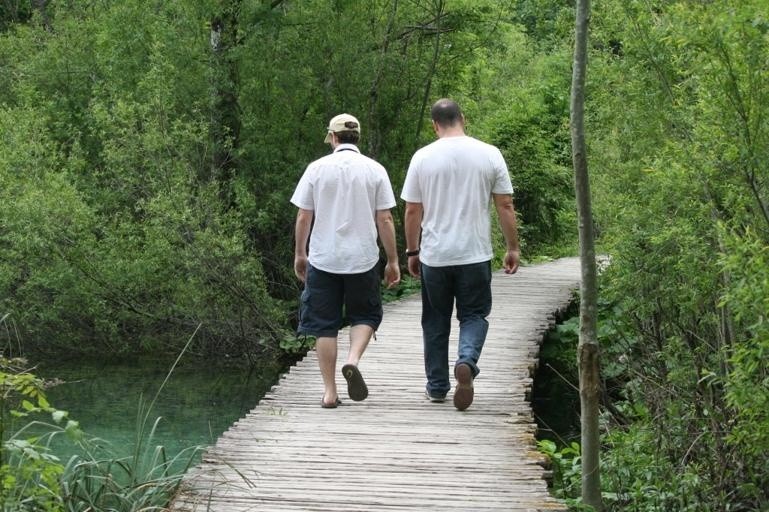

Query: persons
[398,97,523,411]
[289,112,401,407]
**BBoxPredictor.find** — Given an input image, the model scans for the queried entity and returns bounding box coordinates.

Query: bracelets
[405,248,421,258]
[506,249,521,251]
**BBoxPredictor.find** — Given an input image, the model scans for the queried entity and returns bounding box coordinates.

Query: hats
[323,113,360,144]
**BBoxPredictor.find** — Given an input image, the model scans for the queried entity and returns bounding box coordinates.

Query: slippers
[342,364,368,402]
[320,395,339,408]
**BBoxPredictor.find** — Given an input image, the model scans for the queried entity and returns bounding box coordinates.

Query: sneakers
[424,389,447,402]
[452,363,475,411]
